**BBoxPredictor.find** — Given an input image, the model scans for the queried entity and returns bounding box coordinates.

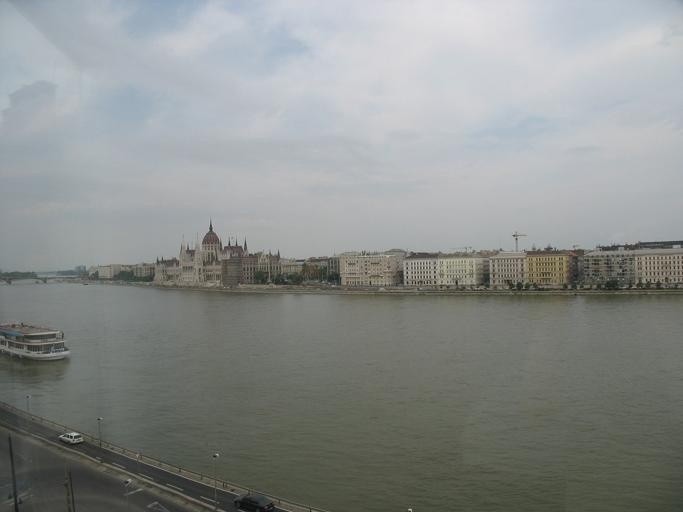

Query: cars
[59,431,85,445]
[232,494,276,511]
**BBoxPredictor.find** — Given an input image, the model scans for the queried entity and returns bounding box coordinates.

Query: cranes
[512,232,527,250]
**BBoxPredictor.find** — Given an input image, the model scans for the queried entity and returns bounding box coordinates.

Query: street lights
[211,451,220,500]
[97,417,104,448]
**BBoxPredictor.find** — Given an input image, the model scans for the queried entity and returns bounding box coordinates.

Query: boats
[0,320,71,360]
